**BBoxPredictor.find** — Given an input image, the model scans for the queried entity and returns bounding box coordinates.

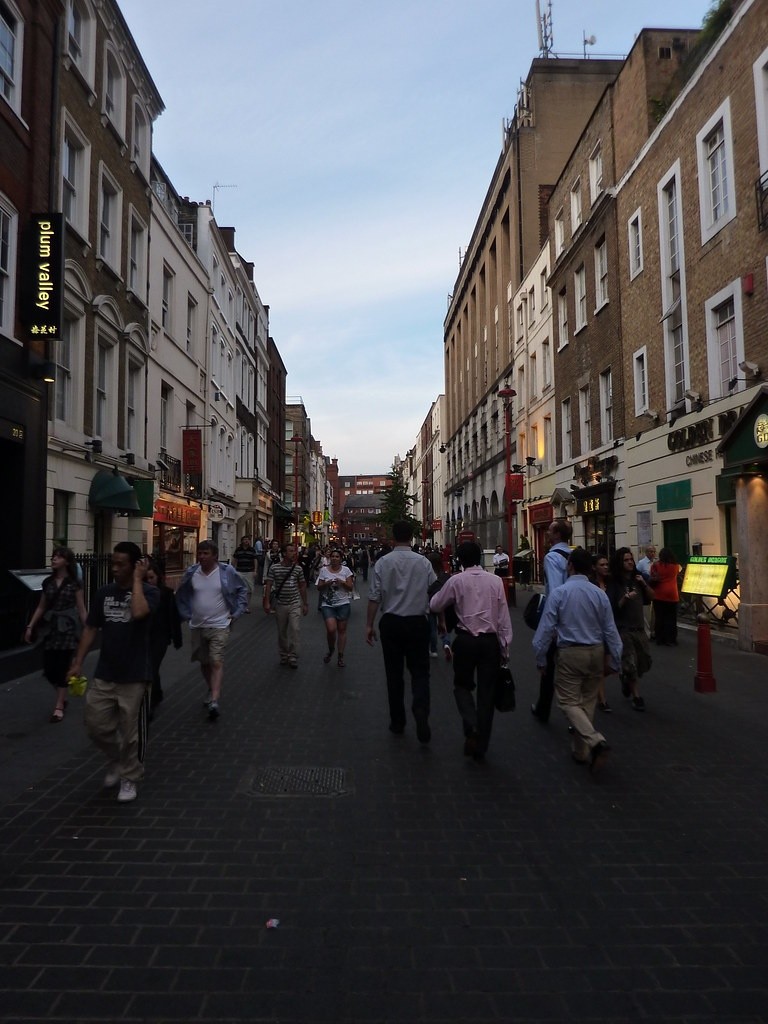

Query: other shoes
[600,702,611,711]
[288,656,298,668]
[573,751,594,765]
[279,658,287,664]
[432,653,438,657]
[633,696,645,710]
[51,708,64,722]
[210,702,219,718]
[619,673,631,698]
[203,697,211,705]
[591,740,609,779]
[444,644,451,660]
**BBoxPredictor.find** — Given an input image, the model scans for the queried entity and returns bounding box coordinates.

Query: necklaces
[331,564,341,571]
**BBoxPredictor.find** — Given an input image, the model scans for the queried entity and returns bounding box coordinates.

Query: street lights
[421,479,429,553]
[497,384,518,608]
[291,433,303,564]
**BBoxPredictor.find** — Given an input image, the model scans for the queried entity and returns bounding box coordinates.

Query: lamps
[85,439,103,452]
[120,452,137,464]
[739,359,759,378]
[62,447,93,464]
[440,442,450,453]
[685,390,699,401]
[636,426,654,440]
[513,464,526,473]
[644,410,657,420]
[670,411,685,427]
[94,459,119,476]
[696,393,734,412]
[728,378,762,390]
[526,456,542,474]
[148,460,170,472]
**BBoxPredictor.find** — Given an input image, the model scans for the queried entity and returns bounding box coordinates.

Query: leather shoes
[568,726,576,733]
[531,703,550,723]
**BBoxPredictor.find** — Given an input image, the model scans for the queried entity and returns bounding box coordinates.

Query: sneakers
[118,777,137,801]
[103,763,120,787]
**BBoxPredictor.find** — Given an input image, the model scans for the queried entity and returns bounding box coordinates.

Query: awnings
[88,468,141,511]
[274,500,306,522]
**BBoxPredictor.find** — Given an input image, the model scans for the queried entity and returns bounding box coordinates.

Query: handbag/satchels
[523,594,548,630]
[264,590,278,616]
[496,663,516,712]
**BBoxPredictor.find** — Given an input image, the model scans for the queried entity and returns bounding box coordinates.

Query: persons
[427,554,452,661]
[366,519,448,742]
[25,548,88,722]
[315,551,354,668]
[532,549,623,765]
[262,543,308,669]
[531,520,573,724]
[175,541,252,716]
[429,542,513,763]
[230,535,510,614]
[143,565,182,728]
[69,542,159,800]
[587,547,683,709]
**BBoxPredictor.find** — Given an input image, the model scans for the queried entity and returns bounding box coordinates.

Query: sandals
[324,652,334,662]
[338,653,345,666]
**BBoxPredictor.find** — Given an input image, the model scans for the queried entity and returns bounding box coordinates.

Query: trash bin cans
[500,576,516,607]
[512,557,530,584]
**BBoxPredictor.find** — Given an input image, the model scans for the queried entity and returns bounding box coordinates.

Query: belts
[567,643,592,647]
[618,626,644,633]
